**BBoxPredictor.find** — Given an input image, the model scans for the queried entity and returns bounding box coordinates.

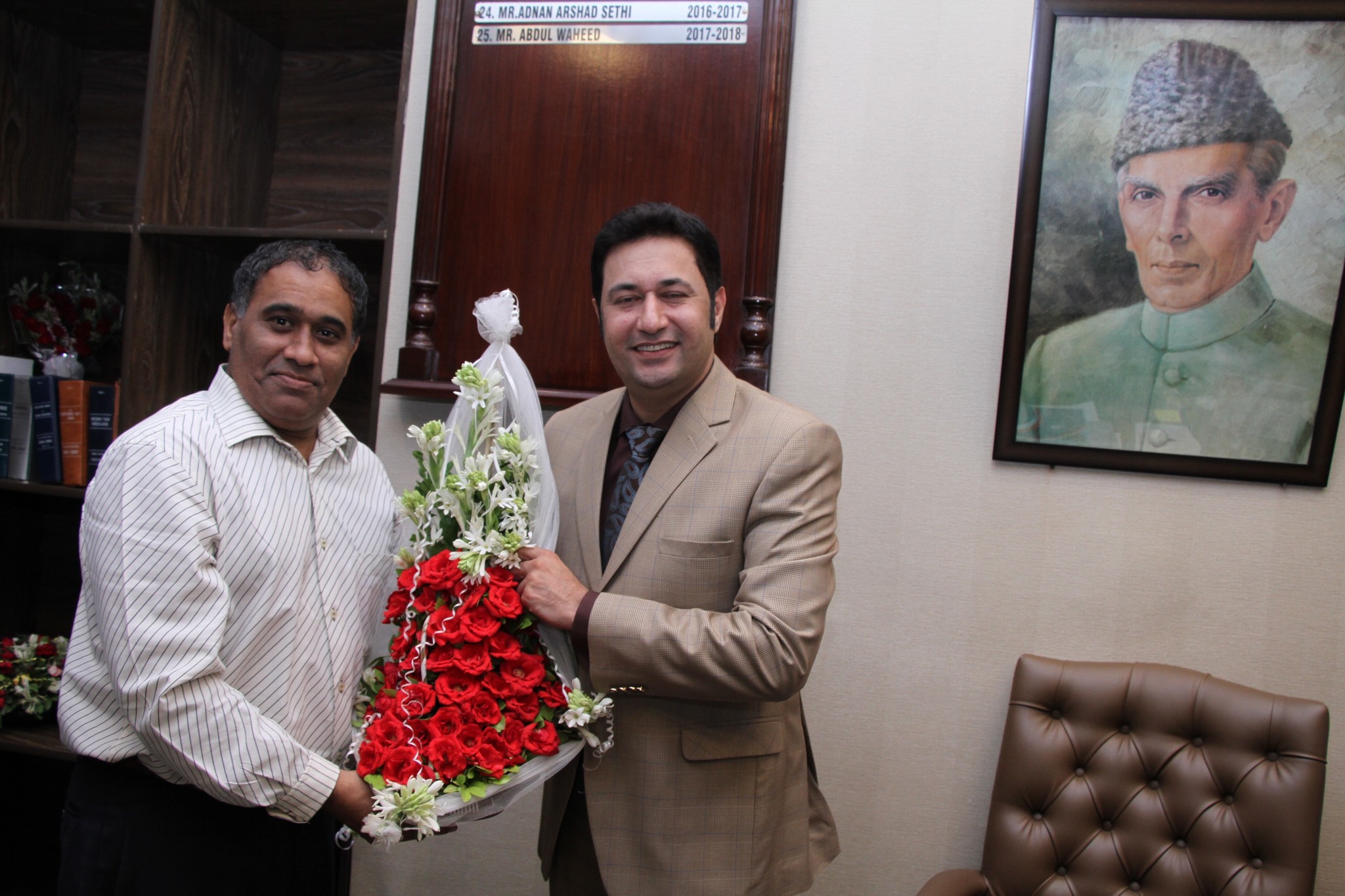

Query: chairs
[916,654,1329,896]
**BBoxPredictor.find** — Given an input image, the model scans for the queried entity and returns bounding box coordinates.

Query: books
[0,372,120,487]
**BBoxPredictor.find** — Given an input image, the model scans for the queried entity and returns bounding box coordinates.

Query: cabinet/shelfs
[0,0,410,896]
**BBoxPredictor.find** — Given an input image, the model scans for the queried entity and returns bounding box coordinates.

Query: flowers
[10,270,125,374]
[342,359,615,850]
[0,635,70,725]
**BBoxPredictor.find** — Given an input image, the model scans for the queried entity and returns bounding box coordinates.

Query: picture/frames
[993,0,1345,487]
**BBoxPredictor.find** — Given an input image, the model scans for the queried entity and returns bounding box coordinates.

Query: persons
[508,202,844,896]
[56,237,405,896]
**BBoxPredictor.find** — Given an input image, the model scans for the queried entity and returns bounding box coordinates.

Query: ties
[602,426,665,575]
